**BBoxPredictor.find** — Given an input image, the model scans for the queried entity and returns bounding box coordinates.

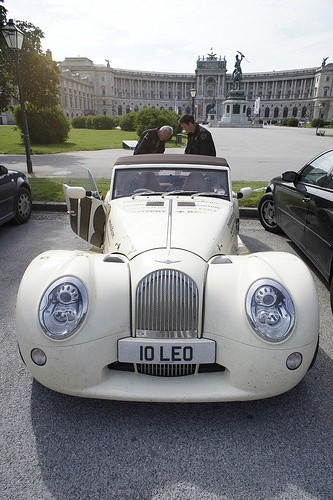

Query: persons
[178,113,216,157]
[133,126,173,156]
[234,55,244,73]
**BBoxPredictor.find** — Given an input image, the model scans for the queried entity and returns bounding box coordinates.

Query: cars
[257,147,333,315]
[0,164,33,226]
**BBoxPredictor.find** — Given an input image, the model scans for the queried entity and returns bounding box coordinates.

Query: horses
[231,69,241,90]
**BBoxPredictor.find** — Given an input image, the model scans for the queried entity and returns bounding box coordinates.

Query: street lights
[190,87,197,116]
[0,19,33,173]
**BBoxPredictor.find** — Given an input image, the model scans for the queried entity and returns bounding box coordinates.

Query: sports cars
[15,155,320,403]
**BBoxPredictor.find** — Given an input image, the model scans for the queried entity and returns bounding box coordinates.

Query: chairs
[138,172,161,192]
[182,172,208,192]
